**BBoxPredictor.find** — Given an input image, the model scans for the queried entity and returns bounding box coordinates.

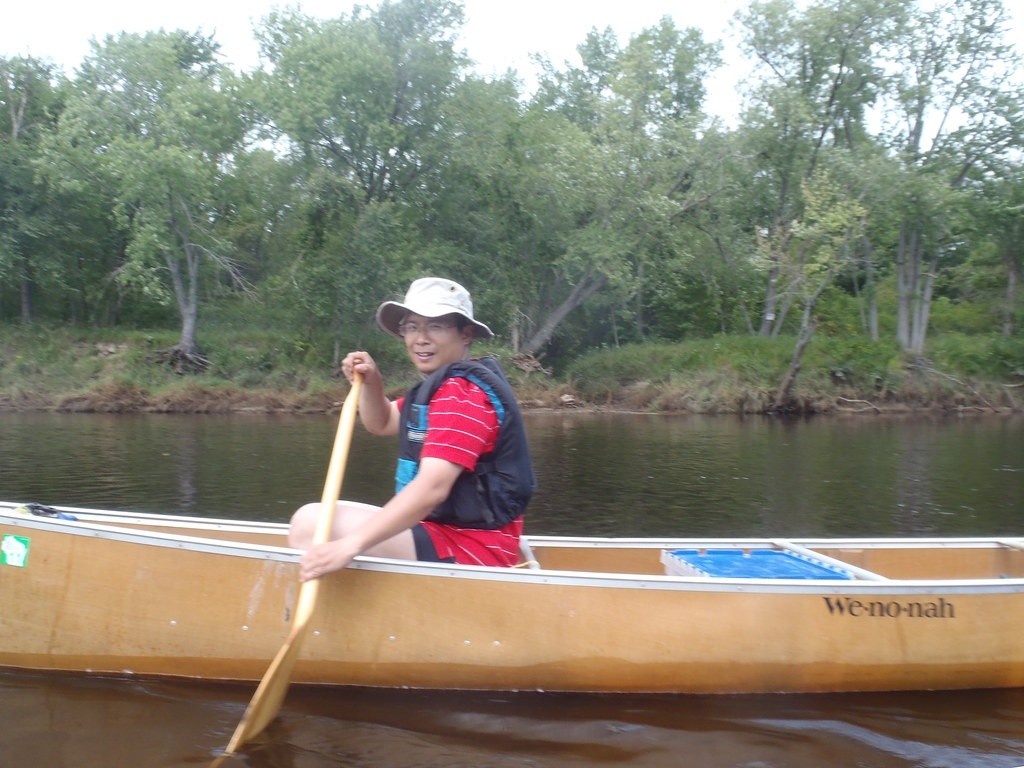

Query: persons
[288,277,535,583]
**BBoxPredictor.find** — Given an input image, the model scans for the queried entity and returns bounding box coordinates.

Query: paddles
[216,357,362,767]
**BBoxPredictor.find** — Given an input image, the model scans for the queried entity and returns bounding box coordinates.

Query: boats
[1,500,1023,704]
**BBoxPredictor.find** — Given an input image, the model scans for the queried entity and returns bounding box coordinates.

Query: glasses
[397,321,462,337]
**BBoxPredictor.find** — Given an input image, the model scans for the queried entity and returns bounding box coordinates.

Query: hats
[377,277,495,341]
